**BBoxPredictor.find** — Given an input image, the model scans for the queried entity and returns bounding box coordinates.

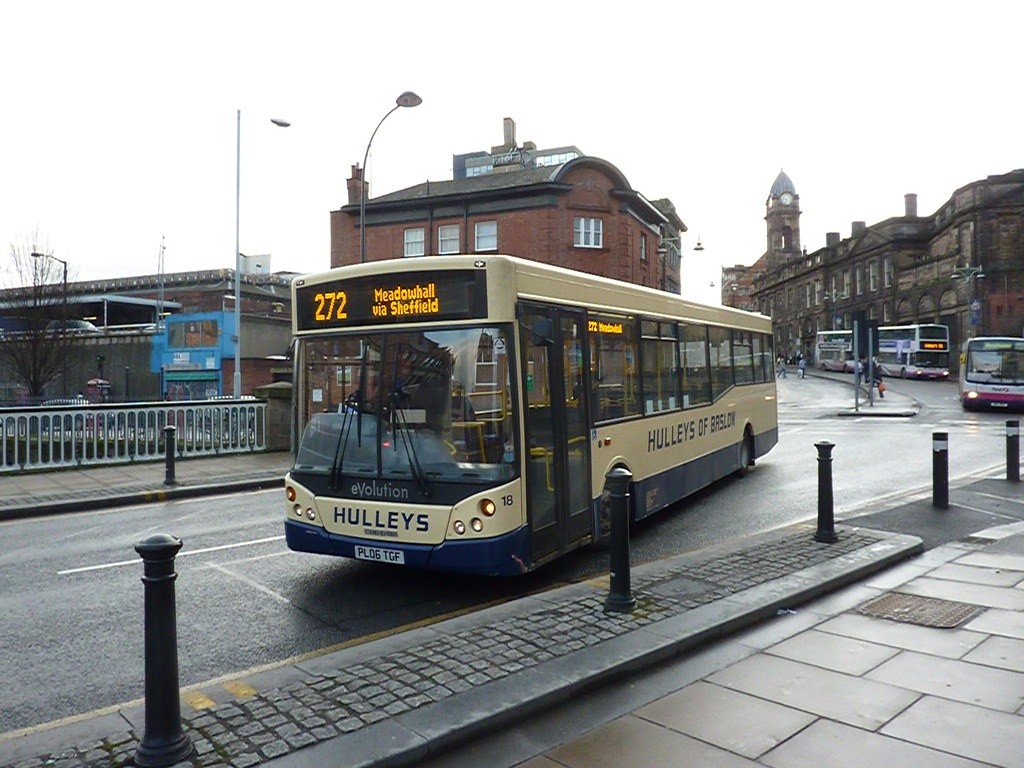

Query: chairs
[452,364,764,455]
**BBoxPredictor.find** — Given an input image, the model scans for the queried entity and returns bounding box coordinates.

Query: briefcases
[797,369,804,377]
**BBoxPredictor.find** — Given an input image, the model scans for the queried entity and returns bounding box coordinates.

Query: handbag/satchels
[879,383,886,392]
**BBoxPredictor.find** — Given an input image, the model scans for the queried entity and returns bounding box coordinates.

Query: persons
[798,356,809,380]
[778,358,787,378]
[857,356,884,399]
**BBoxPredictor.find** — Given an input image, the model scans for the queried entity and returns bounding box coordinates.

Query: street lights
[360,92,422,263]
[234,105,290,400]
[32,251,68,396]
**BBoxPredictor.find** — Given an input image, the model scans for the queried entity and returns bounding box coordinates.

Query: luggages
[873,387,880,402]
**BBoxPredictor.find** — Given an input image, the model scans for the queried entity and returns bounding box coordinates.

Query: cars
[40,399,89,429]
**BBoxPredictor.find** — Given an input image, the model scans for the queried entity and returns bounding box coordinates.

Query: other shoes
[777,375,788,378]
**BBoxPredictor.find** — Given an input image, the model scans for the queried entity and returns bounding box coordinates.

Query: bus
[285,254,778,580]
[815,324,950,380]
[959,338,1024,414]
